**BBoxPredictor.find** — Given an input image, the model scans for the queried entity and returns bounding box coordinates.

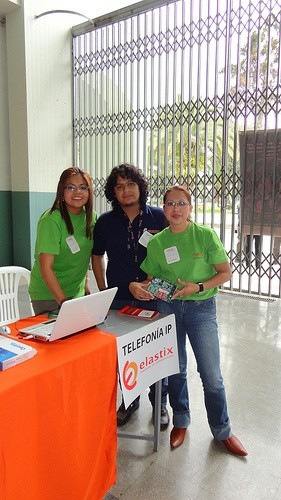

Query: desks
[0,310,180,500]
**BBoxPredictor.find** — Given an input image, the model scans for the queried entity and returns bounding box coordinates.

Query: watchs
[196,282,204,295]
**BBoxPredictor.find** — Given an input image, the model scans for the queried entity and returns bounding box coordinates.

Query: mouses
[0,325,11,335]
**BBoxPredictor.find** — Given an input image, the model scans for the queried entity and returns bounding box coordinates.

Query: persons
[92,164,170,430]
[28,168,97,316]
[129,186,247,457]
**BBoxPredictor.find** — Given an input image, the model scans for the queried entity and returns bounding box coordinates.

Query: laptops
[19,286,118,342]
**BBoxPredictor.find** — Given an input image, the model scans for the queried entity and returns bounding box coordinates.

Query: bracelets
[85,292,89,295]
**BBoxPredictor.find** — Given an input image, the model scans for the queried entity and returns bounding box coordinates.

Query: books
[117,305,159,321]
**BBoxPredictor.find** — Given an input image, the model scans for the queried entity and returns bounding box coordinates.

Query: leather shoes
[170,428,186,448]
[117,399,139,427]
[223,433,247,456]
[153,405,169,430]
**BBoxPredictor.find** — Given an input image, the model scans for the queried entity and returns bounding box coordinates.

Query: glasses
[163,201,189,207]
[63,184,88,192]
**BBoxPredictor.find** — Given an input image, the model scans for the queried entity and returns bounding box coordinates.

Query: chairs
[1,265,36,325]
